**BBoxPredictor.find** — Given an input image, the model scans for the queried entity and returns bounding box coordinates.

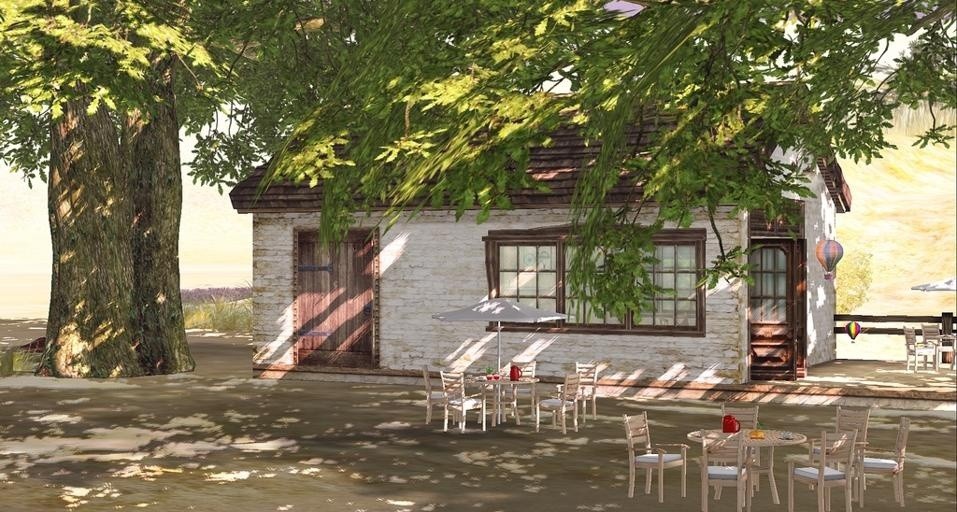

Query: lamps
[423,361,598,433]
[904,324,957,372]
[624,401,911,512]
